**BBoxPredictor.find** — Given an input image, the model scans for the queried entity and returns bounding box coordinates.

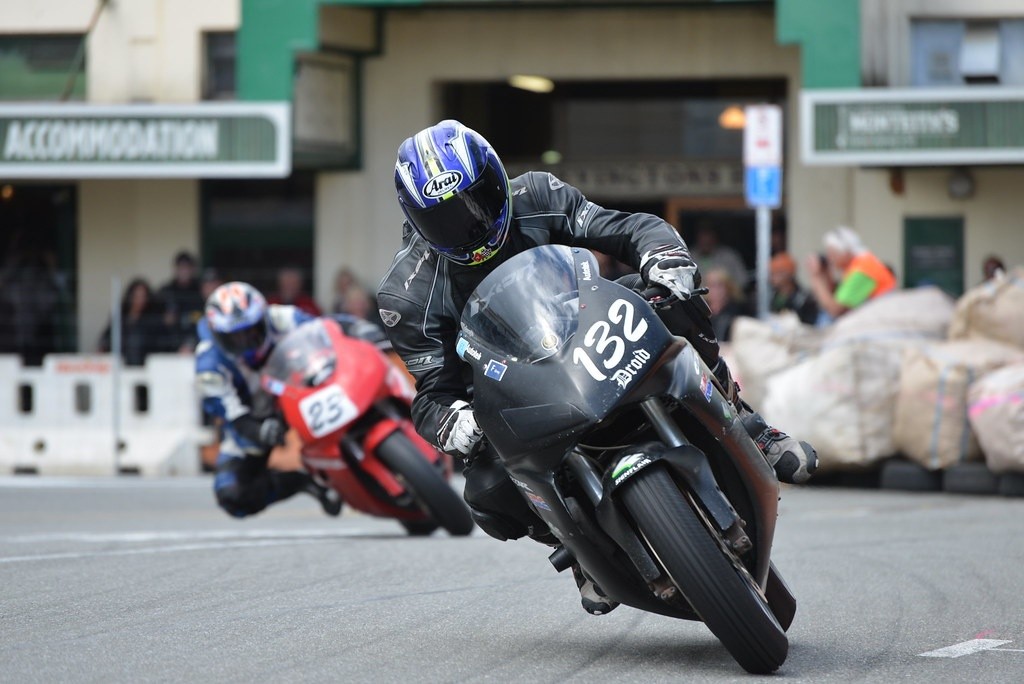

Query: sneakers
[571,564,620,616]
[754,425,818,484]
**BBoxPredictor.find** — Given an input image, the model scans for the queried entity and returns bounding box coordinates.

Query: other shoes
[306,473,340,518]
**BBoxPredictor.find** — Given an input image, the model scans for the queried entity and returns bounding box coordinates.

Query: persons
[983,256,1003,280]
[685,225,895,341]
[197,283,342,518]
[377,120,819,616]
[98,252,375,366]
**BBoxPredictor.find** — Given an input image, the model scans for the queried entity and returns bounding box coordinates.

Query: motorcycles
[262,318,477,543]
[456,244,797,676]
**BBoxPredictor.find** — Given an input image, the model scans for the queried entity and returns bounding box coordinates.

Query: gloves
[437,399,489,459]
[640,243,701,301]
[258,417,286,449]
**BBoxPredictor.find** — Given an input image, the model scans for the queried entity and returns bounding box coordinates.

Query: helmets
[394,119,512,268]
[206,281,273,367]
